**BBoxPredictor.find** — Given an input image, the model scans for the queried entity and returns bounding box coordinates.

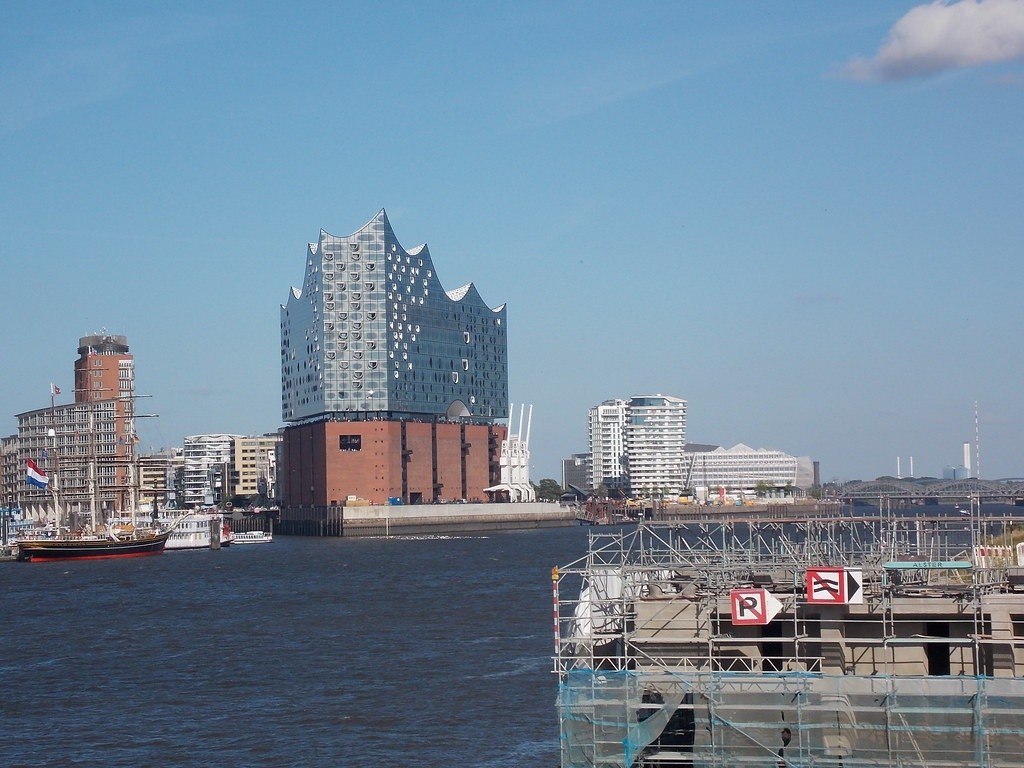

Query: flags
[118,437,124,444]
[134,436,139,442]
[27,458,49,489]
[52,384,61,397]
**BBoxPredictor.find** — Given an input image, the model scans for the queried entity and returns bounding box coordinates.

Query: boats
[958,508,970,516]
[16,345,194,562]
[954,503,959,509]
[229,531,273,543]
[107,505,235,550]
